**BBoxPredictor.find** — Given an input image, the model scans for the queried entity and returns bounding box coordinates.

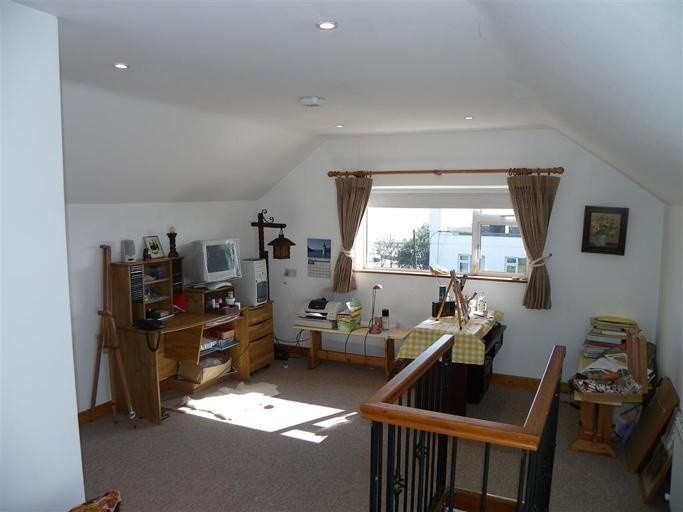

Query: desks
[571,352,620,458]
[292,325,411,382]
[413,312,503,405]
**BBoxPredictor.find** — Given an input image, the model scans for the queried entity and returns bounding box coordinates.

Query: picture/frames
[143,235,165,258]
[581,204,630,256]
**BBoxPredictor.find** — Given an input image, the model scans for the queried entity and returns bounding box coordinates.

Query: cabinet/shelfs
[108,258,274,426]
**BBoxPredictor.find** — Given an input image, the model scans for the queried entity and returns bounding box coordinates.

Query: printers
[295,297,345,329]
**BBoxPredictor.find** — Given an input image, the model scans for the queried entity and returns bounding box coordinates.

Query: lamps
[369,285,383,334]
[250,209,295,259]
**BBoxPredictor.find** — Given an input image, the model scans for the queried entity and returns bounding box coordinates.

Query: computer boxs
[233,258,268,307]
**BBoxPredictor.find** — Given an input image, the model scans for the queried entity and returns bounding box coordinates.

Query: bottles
[477,291,487,315]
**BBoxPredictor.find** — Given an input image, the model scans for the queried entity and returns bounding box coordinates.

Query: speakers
[121,240,136,262]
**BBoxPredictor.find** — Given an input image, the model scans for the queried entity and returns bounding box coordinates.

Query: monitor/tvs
[177,238,242,291]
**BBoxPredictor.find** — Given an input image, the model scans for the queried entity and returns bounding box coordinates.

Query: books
[582,315,639,360]
